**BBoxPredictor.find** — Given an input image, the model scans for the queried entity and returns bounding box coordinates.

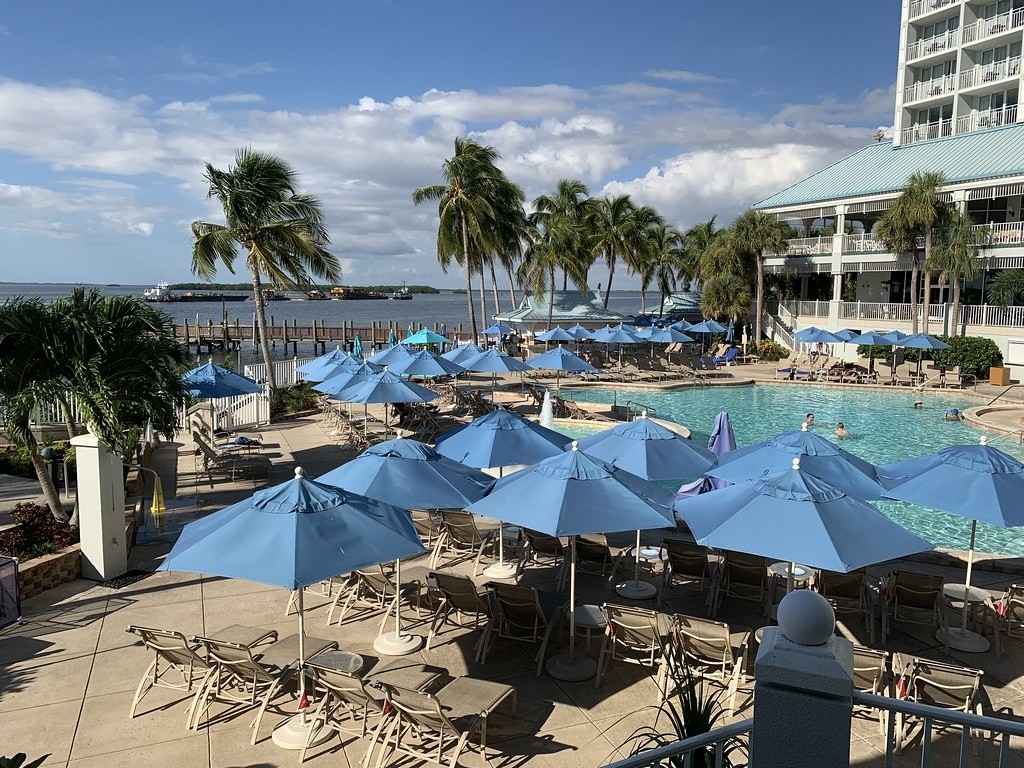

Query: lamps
[882,284,888,291]
[861,281,869,288]
[1008,206,1015,218]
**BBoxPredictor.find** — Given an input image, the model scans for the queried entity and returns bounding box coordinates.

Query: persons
[835,422,848,434]
[914,402,922,406]
[945,409,959,419]
[502,334,507,347]
[807,414,814,421]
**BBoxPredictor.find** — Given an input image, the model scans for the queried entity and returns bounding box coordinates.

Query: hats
[951,409,958,414]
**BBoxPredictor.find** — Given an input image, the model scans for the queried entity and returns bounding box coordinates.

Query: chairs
[192,412,272,487]
[534,344,762,386]
[312,376,617,460]
[775,350,964,389]
[124,509,1024,768]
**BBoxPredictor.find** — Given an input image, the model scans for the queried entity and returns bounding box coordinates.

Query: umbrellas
[154,317,1024,722]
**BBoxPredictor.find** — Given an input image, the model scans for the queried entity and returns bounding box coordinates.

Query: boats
[260,288,291,302]
[140,277,250,303]
[388,280,414,300]
[304,275,332,301]
[329,282,388,300]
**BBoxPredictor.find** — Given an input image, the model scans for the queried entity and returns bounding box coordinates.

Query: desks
[769,562,816,593]
[308,649,364,718]
[218,445,242,455]
[944,583,996,637]
[498,525,522,554]
[566,604,607,657]
[911,377,925,387]
[859,373,876,385]
